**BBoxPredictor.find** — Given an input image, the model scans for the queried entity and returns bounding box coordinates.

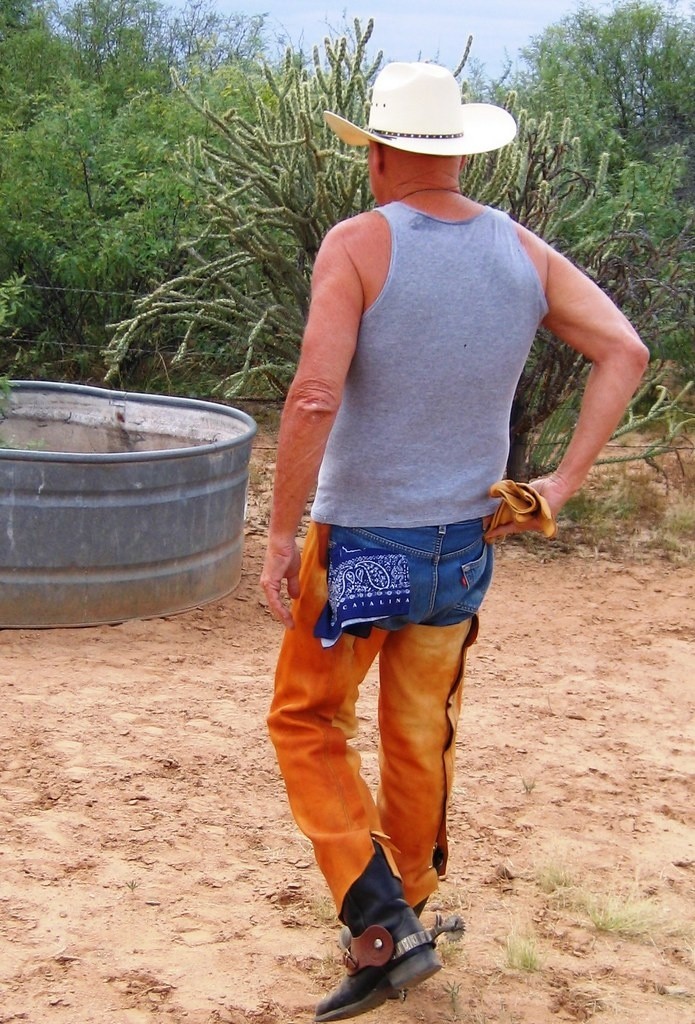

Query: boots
[314,840,440,1023]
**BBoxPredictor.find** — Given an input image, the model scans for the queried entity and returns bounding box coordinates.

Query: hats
[324,62,518,156]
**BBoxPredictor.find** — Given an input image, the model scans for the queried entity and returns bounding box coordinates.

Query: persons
[259,61,650,1022]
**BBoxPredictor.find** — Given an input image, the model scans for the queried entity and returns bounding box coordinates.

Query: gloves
[484,480,556,544]
